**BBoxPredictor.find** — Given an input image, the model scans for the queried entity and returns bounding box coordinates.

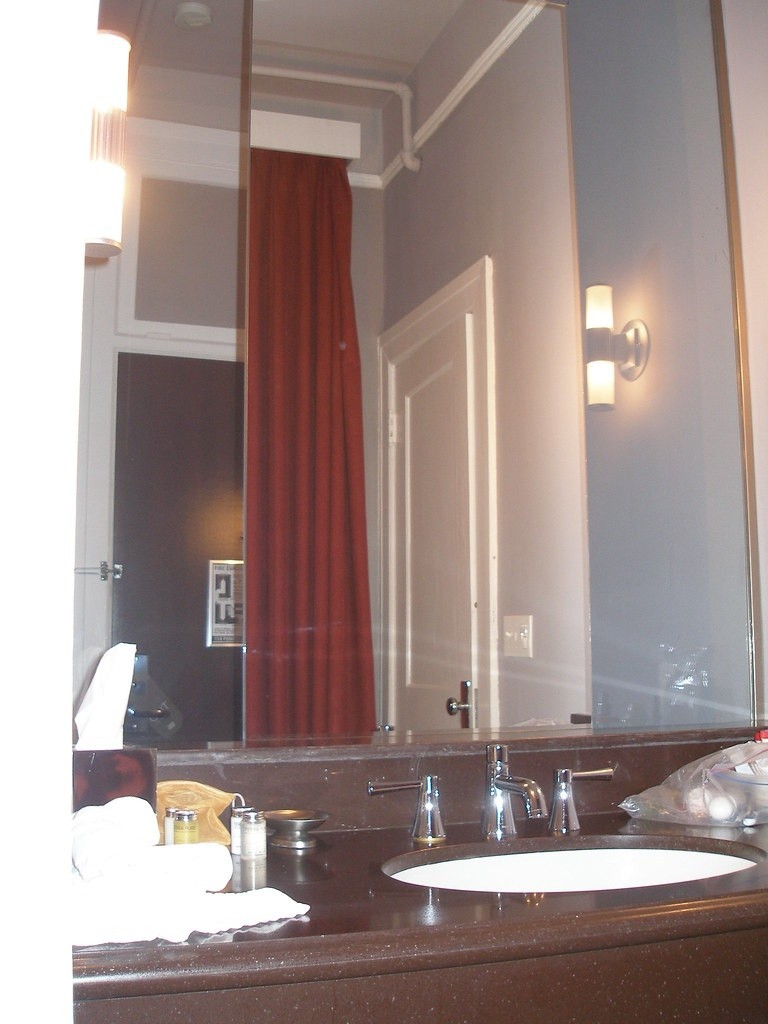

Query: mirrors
[72,0,768,750]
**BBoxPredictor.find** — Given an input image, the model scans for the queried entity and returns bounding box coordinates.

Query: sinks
[380,831,768,897]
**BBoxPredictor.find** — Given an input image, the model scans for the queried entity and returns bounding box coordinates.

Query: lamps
[583,282,649,409]
[85,27,128,258]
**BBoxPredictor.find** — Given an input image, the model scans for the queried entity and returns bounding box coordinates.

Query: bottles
[241,811,267,861]
[231,806,253,854]
[232,855,241,892]
[175,810,199,844]
[241,860,268,890]
[164,807,187,845]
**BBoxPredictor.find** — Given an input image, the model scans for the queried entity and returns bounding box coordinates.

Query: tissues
[73,640,156,821]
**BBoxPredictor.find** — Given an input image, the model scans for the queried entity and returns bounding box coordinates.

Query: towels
[73,786,311,947]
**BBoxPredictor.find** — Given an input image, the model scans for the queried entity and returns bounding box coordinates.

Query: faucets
[482,741,548,838]
